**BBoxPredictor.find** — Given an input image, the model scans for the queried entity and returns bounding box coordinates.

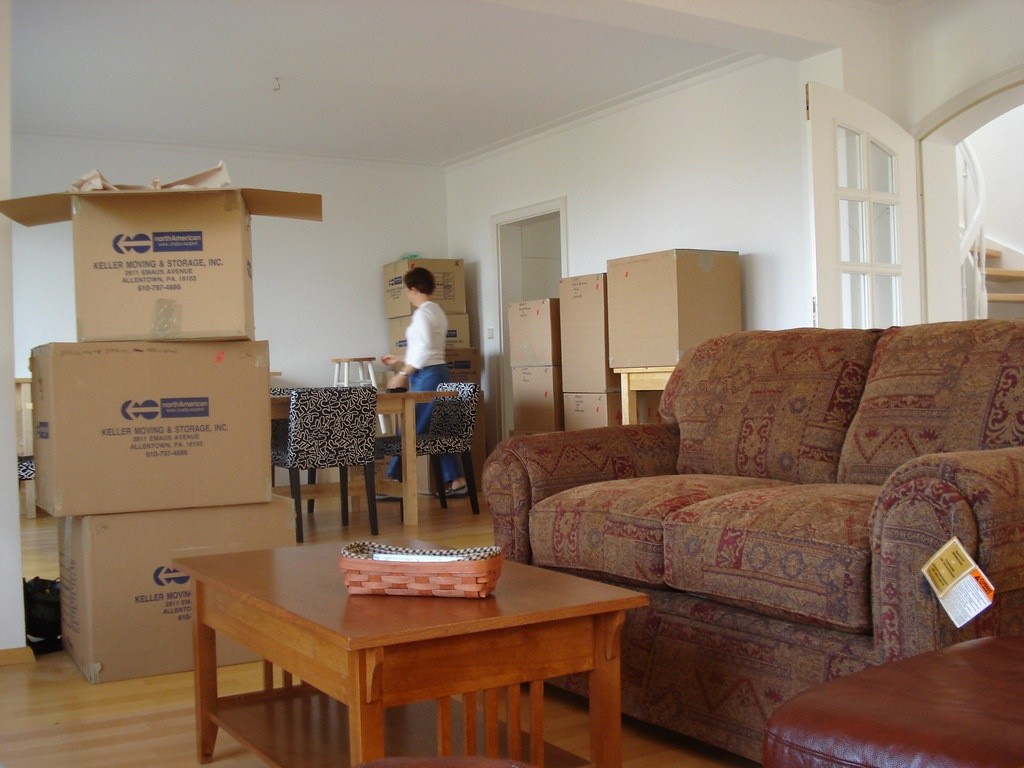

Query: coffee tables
[161,536,650,768]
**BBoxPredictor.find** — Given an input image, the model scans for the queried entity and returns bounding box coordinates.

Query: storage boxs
[0,162,323,684]
[318,256,487,498]
[508,249,743,444]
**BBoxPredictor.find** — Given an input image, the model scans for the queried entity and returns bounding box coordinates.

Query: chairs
[376,381,481,523]
[270,388,380,544]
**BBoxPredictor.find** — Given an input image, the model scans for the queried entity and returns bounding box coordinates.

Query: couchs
[482,316,1024,765]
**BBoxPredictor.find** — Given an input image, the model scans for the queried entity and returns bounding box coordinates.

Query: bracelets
[398,370,410,377]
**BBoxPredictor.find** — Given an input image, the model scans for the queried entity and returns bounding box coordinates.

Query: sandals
[376,494,391,499]
[436,484,468,498]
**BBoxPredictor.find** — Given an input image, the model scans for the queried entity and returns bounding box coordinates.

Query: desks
[272,391,459,527]
[612,366,676,425]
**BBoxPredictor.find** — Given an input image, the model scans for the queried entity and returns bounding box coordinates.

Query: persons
[374,267,467,502]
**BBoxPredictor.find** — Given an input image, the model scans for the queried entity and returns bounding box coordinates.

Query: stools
[333,358,387,435]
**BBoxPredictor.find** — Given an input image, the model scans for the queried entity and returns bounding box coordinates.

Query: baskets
[340,540,503,598]
